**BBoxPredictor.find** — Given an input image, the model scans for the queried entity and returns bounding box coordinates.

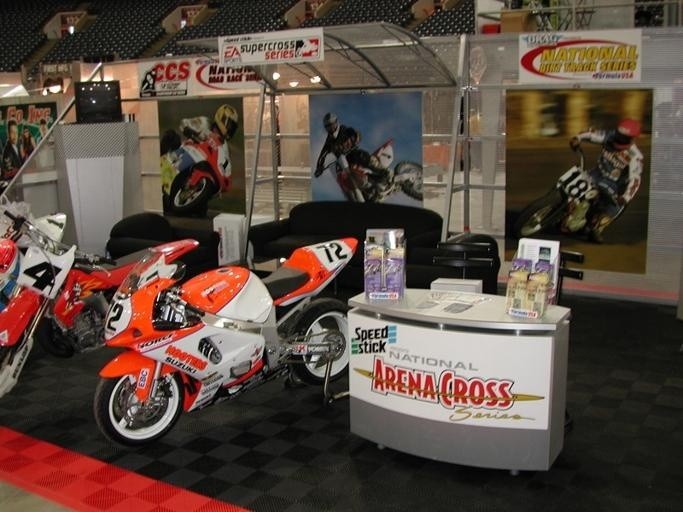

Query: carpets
[0,291,681,511]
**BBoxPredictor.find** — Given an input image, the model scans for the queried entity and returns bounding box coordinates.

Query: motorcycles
[320,140,424,204]
[514,143,628,242]
[0,212,197,401]
[162,129,234,217]
[95,236,357,450]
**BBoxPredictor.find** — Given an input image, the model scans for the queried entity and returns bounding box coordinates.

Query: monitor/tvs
[73,80,123,122]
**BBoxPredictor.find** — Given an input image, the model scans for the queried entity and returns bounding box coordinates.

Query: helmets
[612,118,640,150]
[323,112,341,140]
[214,104,239,141]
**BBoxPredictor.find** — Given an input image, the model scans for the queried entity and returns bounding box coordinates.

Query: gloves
[569,136,580,152]
[184,126,201,140]
[614,196,628,210]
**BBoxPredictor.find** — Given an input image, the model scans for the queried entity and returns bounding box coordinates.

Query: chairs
[105,211,219,278]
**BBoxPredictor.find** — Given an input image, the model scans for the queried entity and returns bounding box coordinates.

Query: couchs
[250,201,443,290]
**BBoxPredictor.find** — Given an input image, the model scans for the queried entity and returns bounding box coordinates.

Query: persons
[160,102,239,217]
[314,112,392,187]
[2,119,23,171]
[567,116,647,246]
[35,119,50,145]
[19,124,37,163]
